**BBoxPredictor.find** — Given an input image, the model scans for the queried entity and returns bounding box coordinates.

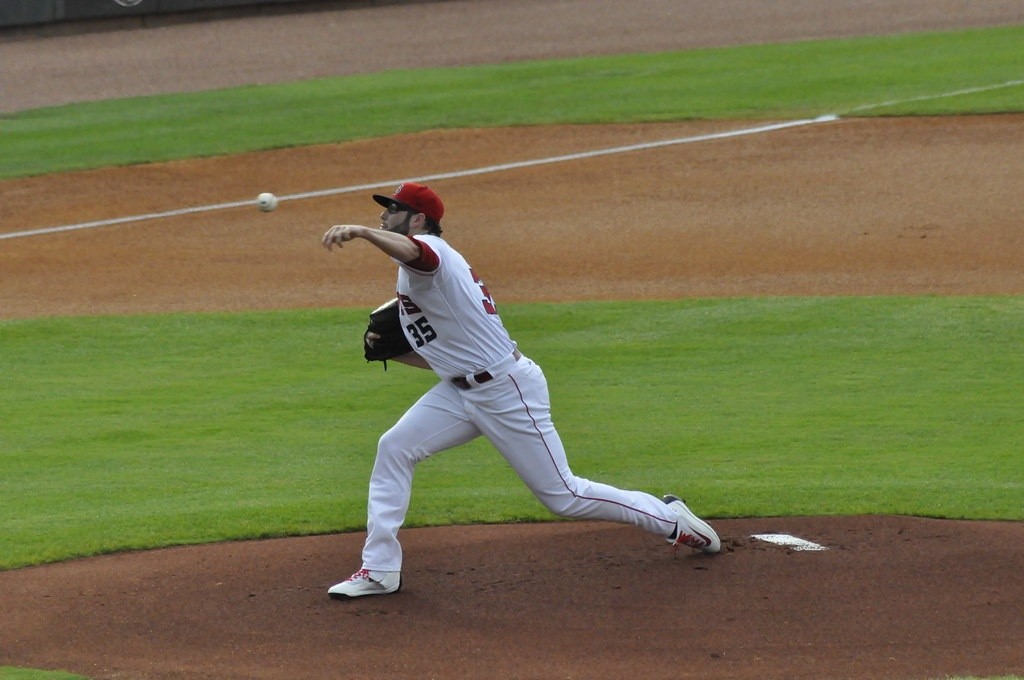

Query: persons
[319,181,721,602]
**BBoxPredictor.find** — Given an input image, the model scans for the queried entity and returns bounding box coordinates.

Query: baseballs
[257,192,279,213]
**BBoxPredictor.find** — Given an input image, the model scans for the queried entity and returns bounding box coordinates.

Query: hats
[373,183,445,224]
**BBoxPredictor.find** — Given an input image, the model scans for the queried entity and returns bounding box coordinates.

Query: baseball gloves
[364,297,414,360]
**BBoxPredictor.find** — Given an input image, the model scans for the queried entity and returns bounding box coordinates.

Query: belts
[450,350,520,390]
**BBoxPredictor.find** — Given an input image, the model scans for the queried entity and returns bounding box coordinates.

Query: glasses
[387,204,408,214]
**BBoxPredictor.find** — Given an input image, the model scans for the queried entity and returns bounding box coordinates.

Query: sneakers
[327,570,402,599]
[660,495,722,555]
